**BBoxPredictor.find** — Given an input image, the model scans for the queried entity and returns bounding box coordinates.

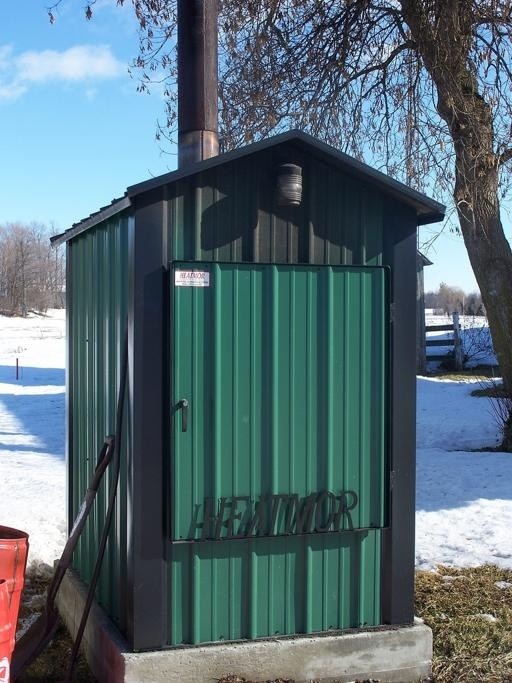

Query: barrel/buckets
[0,525,30,683]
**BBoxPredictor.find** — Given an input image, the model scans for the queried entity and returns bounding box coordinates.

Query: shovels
[10,435,116,681]
